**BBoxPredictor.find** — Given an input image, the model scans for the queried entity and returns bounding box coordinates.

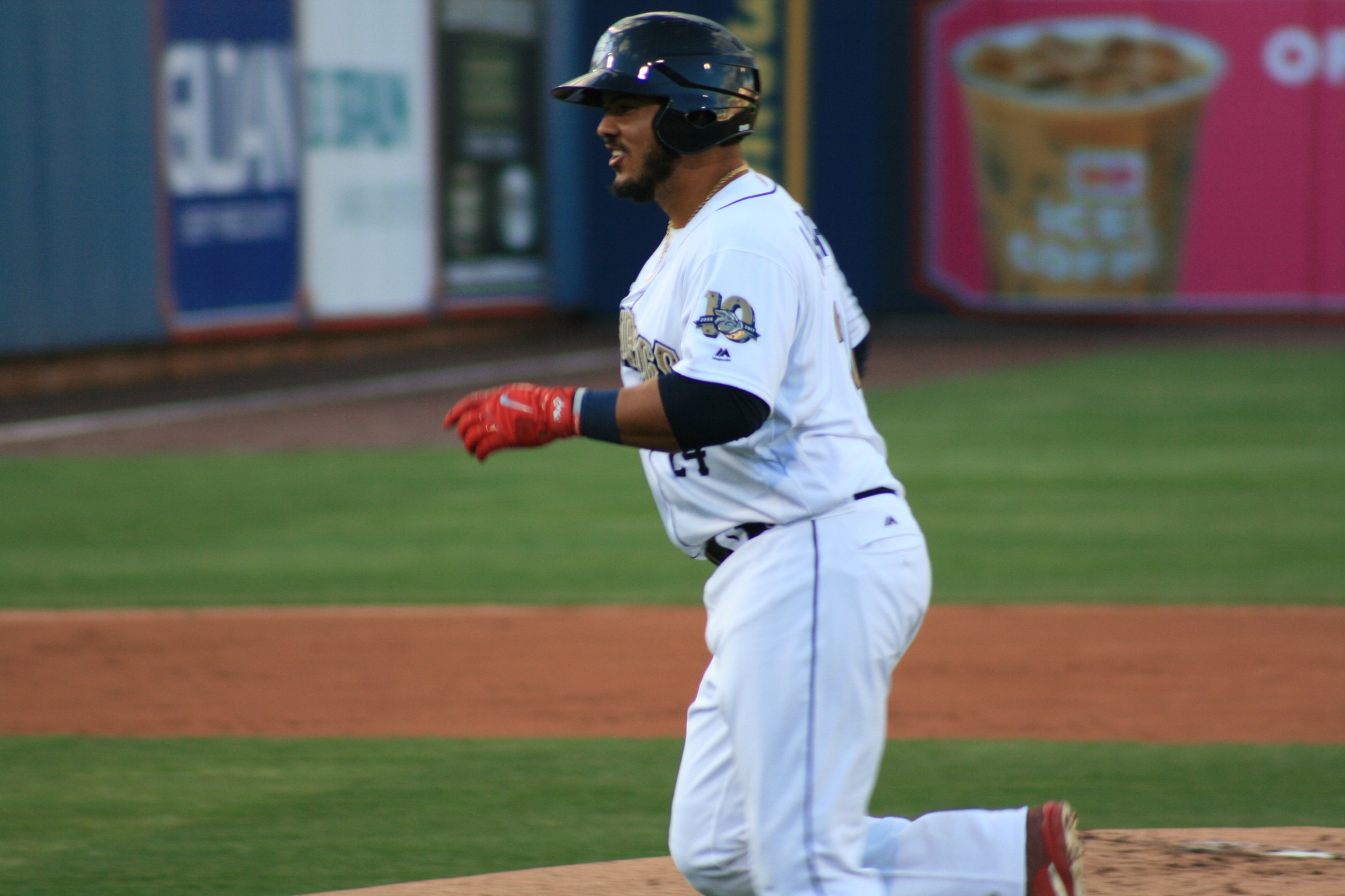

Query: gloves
[442,380,580,462]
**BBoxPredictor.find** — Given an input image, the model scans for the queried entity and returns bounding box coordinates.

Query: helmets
[550,9,763,157]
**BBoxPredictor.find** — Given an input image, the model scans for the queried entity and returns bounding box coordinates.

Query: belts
[701,486,898,567]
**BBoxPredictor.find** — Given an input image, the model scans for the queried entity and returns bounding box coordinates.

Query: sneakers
[1025,799,1089,896]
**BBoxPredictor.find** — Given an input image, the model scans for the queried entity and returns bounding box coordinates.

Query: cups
[951,16,1225,299]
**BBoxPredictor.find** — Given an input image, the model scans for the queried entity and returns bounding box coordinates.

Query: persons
[440,6,1099,896]
